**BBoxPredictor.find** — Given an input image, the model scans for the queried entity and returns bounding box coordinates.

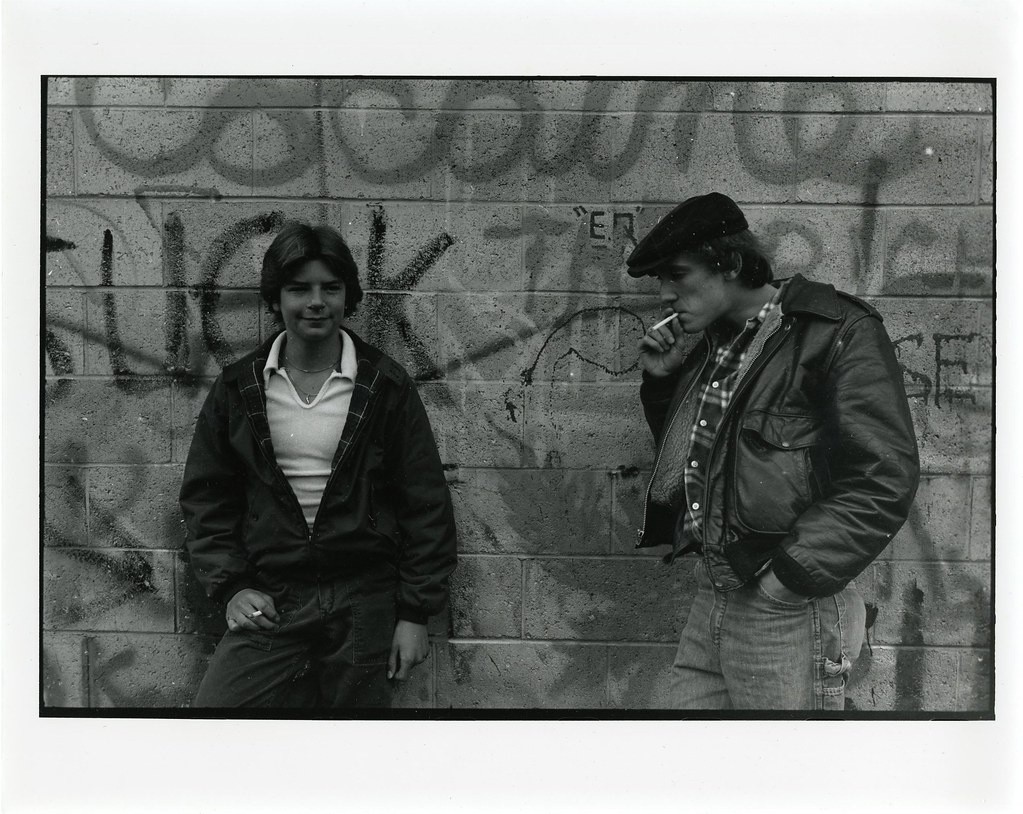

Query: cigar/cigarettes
[648,312,678,332]
[248,610,262,618]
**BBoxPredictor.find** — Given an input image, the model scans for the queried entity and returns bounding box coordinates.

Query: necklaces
[281,357,341,404]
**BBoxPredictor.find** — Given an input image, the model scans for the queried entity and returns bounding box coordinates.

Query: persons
[179,222,457,709]
[627,191,919,709]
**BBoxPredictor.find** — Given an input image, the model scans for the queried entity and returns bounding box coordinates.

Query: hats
[626,192,749,278]
